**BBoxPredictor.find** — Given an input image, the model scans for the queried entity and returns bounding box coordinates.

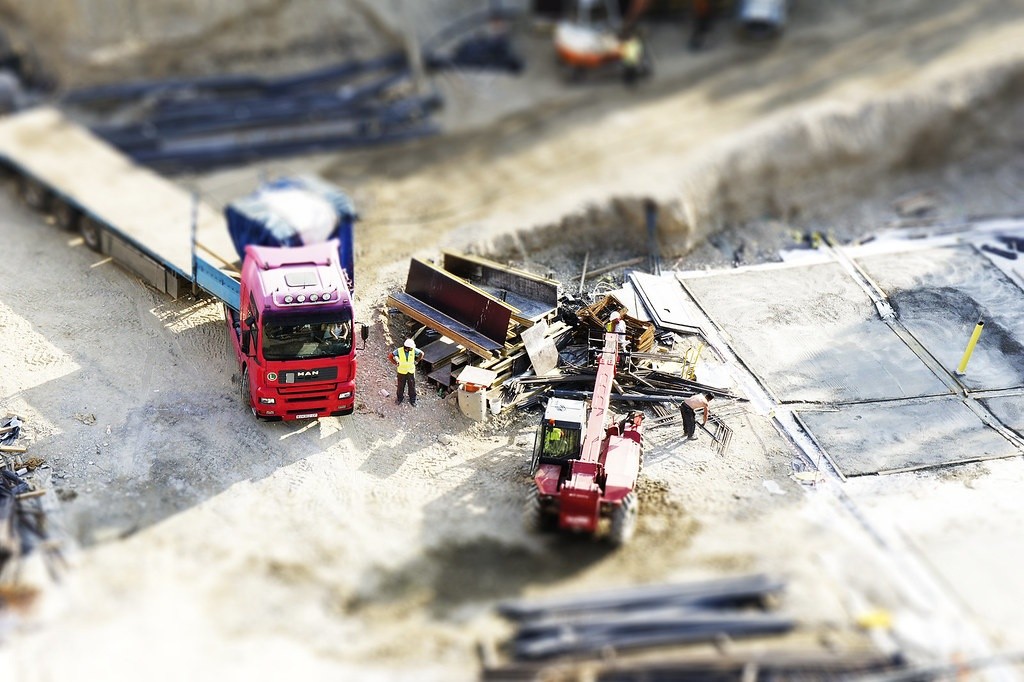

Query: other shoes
[412,402,417,406]
[684,433,688,436]
[687,435,698,439]
[395,398,401,405]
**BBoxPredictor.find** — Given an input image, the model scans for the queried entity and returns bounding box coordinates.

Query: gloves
[414,361,418,365]
[395,362,399,367]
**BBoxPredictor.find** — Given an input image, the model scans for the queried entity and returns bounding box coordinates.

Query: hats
[403,338,416,348]
[708,392,715,398]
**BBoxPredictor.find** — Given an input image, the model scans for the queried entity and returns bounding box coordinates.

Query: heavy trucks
[1,100,370,425]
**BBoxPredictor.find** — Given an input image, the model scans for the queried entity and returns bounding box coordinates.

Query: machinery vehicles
[522,330,646,547]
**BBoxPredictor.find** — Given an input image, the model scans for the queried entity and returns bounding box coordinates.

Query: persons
[387,339,425,407]
[606,311,627,366]
[679,393,714,441]
[544,427,569,457]
[322,322,350,342]
